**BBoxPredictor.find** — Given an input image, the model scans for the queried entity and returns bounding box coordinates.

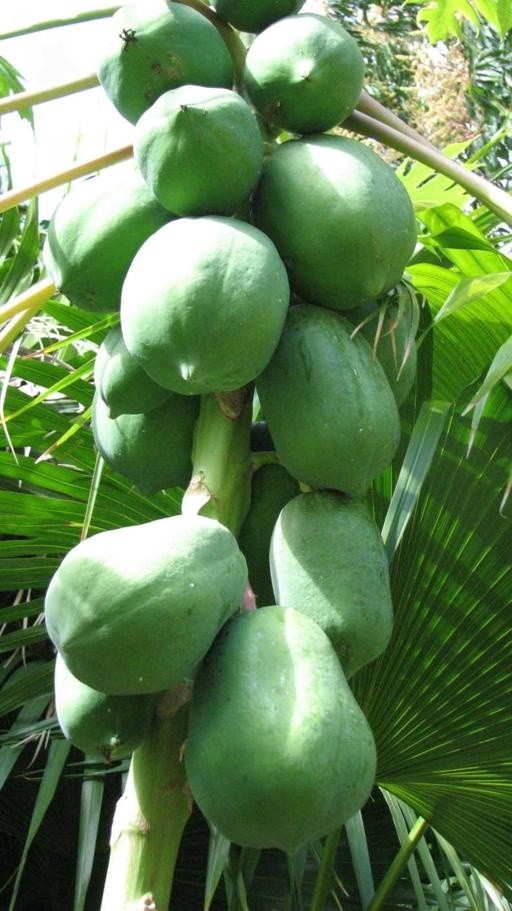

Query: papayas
[43,0,414,856]
